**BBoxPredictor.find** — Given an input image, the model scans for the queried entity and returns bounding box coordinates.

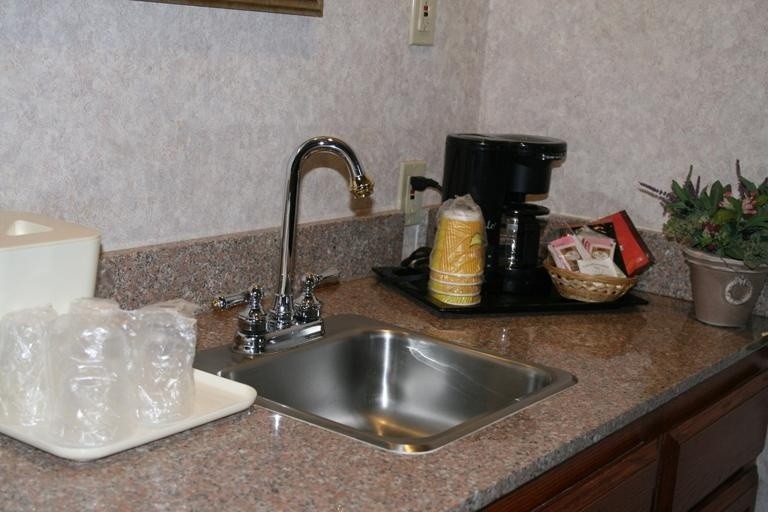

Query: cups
[4,296,185,446]
[427,210,484,306]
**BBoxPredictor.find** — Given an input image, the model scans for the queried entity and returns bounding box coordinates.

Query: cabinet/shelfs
[478,406,659,511]
[658,344,768,512]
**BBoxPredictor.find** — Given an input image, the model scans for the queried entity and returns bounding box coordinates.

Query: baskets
[542,257,639,303]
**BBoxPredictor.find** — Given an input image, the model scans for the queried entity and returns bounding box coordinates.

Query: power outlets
[398,160,426,227]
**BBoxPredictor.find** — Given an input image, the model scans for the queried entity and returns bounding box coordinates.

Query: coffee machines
[435,134,567,293]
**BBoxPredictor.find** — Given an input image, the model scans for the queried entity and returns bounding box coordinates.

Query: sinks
[197,314,576,455]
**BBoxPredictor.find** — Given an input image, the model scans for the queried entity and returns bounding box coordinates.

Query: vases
[681,247,768,329]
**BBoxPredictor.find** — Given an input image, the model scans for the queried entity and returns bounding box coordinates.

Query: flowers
[637,155,768,271]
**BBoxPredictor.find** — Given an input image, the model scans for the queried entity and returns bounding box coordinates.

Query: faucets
[265,135,375,334]
